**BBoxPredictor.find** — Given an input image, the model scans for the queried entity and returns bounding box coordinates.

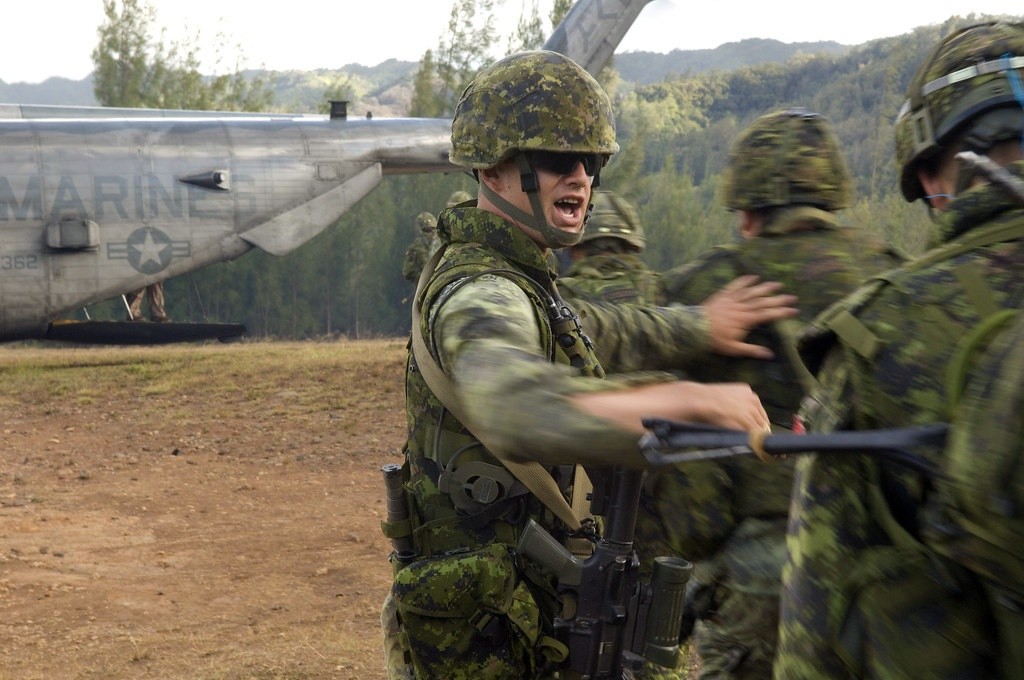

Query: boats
[0,0,656,344]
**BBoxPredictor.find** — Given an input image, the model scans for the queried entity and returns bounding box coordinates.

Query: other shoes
[151,316,171,323]
[127,316,146,321]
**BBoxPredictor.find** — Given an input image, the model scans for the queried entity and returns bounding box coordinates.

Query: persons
[662,113,905,680]
[126,281,170,322]
[383,52,799,679]
[773,21,1024,680]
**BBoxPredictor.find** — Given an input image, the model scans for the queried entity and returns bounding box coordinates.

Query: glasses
[526,153,603,176]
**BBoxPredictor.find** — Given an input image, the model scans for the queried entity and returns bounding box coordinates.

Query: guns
[516,464,694,680]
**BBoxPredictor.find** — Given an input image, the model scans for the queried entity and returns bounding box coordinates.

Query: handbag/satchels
[391,541,569,680]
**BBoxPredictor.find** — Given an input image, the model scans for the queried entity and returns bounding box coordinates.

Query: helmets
[893,99,924,203]
[446,191,473,208]
[913,21,1024,149]
[415,212,438,229]
[570,191,646,254]
[723,111,852,212]
[449,50,620,169]
